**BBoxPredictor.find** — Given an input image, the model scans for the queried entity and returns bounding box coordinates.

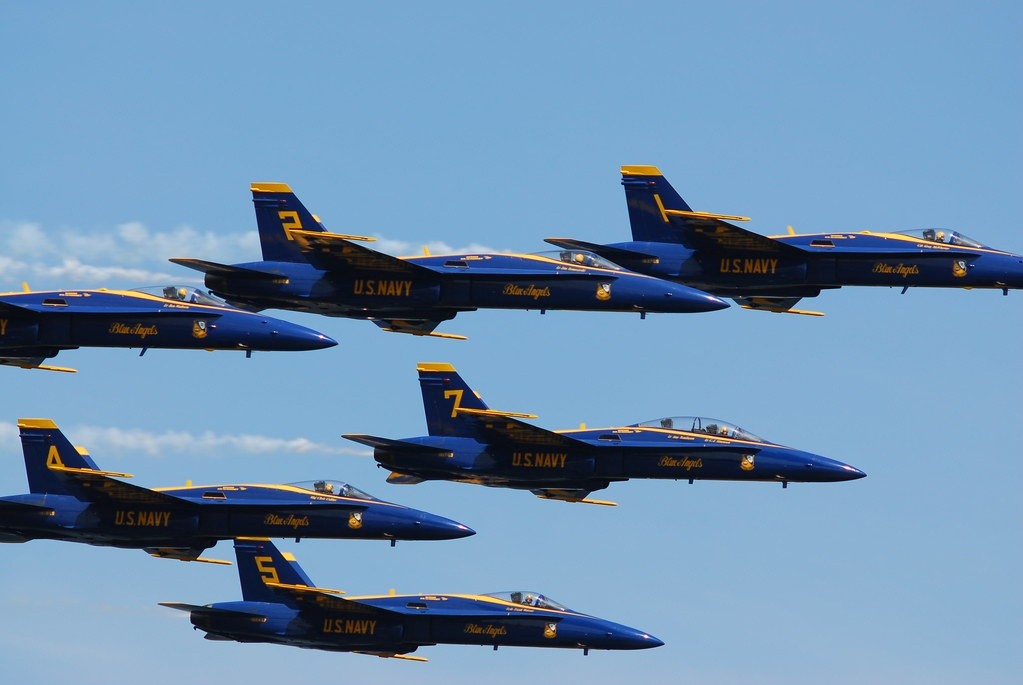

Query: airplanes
[0,285,341,371]
[167,183,732,341]
[543,164,1023,317]
[1,416,476,566]
[159,534,666,662]
[341,361,867,505]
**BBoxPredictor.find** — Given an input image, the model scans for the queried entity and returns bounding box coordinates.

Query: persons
[574,254,584,264]
[936,232,945,242]
[522,594,532,605]
[326,484,334,494]
[176,289,187,301]
[717,427,728,436]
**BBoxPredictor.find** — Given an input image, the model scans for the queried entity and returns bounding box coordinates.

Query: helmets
[720,427,728,432]
[575,254,583,263]
[936,232,945,239]
[326,483,333,490]
[525,594,534,599]
[178,288,187,296]
